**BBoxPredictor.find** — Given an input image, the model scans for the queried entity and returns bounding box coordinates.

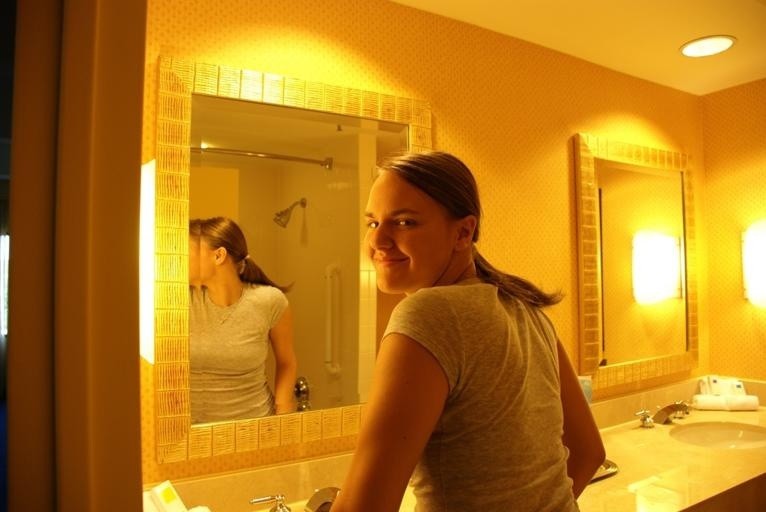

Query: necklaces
[208,285,246,325]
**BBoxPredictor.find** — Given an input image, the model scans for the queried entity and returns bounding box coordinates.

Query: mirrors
[572,132,699,391]
[155,56,433,464]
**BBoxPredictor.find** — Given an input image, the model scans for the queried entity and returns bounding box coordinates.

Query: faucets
[653,402,689,424]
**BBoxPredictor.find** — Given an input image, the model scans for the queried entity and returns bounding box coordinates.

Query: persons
[197,214,299,420]
[323,149,609,511]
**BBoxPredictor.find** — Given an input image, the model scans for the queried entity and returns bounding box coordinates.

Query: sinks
[669,420,766,449]
